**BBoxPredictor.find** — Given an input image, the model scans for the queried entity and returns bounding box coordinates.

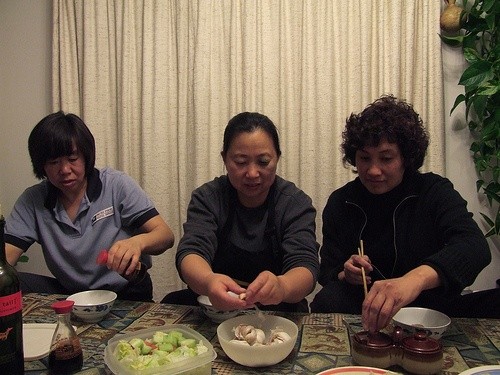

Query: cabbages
[112,339,207,370]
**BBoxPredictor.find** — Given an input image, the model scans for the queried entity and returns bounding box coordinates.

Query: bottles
[95,249,151,288]
[48,301,83,375]
[0,214,25,375]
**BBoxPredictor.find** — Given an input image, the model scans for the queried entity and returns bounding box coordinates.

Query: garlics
[230,324,290,346]
[239,292,247,300]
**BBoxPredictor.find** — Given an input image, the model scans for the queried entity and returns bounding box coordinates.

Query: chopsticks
[22,301,38,317]
[358,240,368,298]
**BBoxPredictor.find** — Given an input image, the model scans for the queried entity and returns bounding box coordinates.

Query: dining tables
[21,292,500,375]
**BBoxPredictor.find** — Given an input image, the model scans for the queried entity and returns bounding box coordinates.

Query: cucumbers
[128,330,197,356]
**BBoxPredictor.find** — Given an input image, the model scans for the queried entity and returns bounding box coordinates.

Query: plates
[315,366,403,375]
[457,364,500,375]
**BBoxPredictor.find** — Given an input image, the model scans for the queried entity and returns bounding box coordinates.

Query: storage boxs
[103,323,218,375]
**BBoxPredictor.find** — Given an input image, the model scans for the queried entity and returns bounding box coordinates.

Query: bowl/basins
[392,307,451,341]
[66,290,117,323]
[197,291,242,323]
[217,315,299,367]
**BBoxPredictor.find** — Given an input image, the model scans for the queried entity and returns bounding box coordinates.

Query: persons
[309,92,493,332]
[6,110,175,301]
[175,112,320,314]
